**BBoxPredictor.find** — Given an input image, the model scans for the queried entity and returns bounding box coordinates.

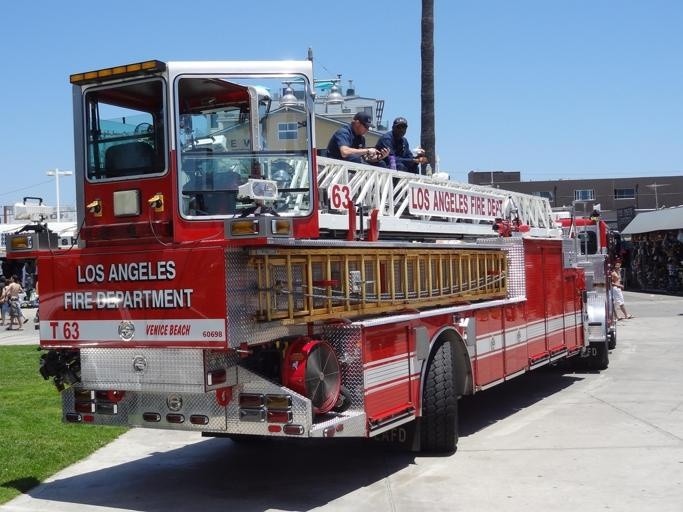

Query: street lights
[45,170,73,222]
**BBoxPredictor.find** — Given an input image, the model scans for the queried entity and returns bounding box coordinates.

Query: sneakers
[617,314,633,321]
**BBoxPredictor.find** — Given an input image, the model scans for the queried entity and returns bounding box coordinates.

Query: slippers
[0,316,29,331]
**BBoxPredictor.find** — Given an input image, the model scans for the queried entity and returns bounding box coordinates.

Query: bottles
[425,163,432,177]
[388,156,397,170]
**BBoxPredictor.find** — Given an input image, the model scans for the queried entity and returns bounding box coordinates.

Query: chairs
[103,143,156,174]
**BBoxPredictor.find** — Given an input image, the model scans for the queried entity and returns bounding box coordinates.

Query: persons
[374,116,430,203]
[0,274,39,332]
[612,298,622,321]
[612,263,632,318]
[327,111,390,213]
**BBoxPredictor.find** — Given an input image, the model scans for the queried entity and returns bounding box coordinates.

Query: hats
[0,276,6,289]
[615,263,622,268]
[392,117,408,129]
[353,112,376,128]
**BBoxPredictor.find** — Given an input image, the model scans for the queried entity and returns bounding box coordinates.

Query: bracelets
[366,149,369,155]
[377,155,381,161]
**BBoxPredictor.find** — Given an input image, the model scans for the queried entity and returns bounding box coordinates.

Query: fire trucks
[36,60,622,457]
